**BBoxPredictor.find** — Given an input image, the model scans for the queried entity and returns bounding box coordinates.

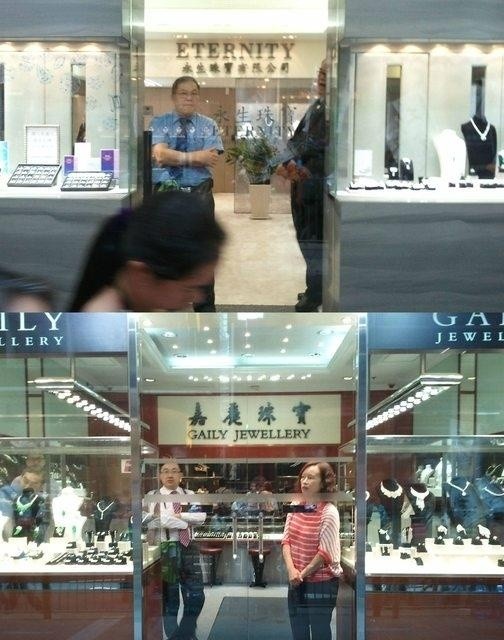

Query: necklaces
[470,118,490,141]
[16,494,39,515]
[448,480,470,497]
[485,487,504,496]
[486,464,501,476]
[410,486,430,510]
[380,480,403,499]
[96,500,114,521]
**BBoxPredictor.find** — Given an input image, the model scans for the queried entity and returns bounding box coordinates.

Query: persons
[281,458,342,640]
[181,476,303,522]
[131,461,205,638]
[72,189,225,313]
[0,453,59,513]
[1,280,55,313]
[281,61,328,313]
[146,77,224,314]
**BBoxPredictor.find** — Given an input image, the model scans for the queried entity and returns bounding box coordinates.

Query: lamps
[28,357,150,434]
[346,354,463,436]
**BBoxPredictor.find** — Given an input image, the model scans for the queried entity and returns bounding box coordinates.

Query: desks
[323,187,502,314]
[334,553,503,639]
[0,542,165,640]
[0,184,133,312]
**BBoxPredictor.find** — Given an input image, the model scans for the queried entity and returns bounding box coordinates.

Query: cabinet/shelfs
[188,520,353,586]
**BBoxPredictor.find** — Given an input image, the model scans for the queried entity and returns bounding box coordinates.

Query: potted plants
[224,124,287,223]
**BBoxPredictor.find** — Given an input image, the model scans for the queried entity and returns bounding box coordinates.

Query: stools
[200,548,223,588]
[245,546,270,590]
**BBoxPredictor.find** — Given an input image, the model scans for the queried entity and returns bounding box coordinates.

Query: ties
[167,119,190,180]
[171,490,193,549]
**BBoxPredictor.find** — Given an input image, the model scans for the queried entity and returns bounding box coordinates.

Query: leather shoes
[293,285,325,315]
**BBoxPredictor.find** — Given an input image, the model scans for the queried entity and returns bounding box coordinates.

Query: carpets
[207,593,297,640]
